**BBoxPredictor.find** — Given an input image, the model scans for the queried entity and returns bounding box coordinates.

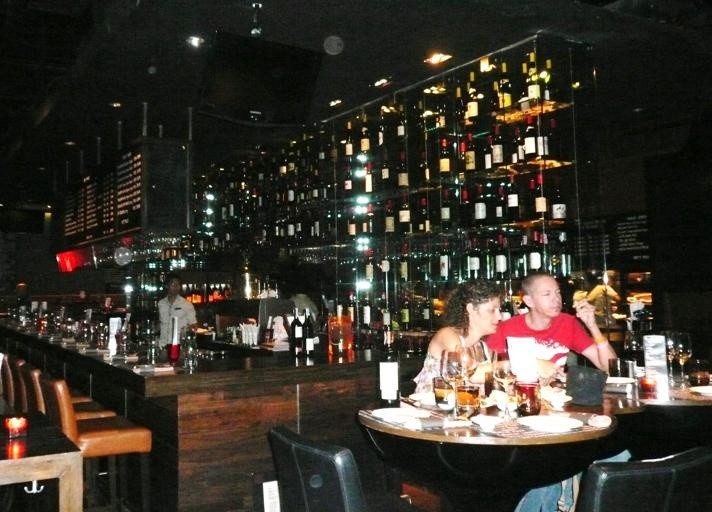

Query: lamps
[243,0,266,41]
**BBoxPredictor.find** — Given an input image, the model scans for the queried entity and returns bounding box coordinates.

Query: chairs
[22,364,117,424]
[265,424,425,511]
[572,446,711,512]
[39,375,154,512]
[9,356,93,414]
[355,408,439,493]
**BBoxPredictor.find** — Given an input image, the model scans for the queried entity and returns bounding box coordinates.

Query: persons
[156,275,197,346]
[283,286,320,324]
[412,277,556,406]
[487,275,618,392]
[585,268,622,327]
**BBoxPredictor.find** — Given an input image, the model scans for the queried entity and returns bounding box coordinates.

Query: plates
[372,407,433,421]
[470,415,505,429]
[516,415,584,433]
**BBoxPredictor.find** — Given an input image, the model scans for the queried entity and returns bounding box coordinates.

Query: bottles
[259,195,263,209]
[458,142,465,172]
[287,140,297,175]
[170,317,181,364]
[279,150,287,178]
[482,237,496,281]
[348,294,357,328]
[549,118,562,159]
[378,293,392,325]
[349,208,358,239]
[454,242,460,280]
[322,176,329,201]
[465,133,479,173]
[456,80,465,120]
[467,72,481,121]
[366,160,375,194]
[416,196,432,234]
[441,189,455,233]
[259,167,264,182]
[419,149,432,188]
[312,170,320,201]
[440,238,453,281]
[470,237,484,280]
[361,118,370,154]
[396,242,413,286]
[485,135,492,172]
[362,297,372,329]
[436,82,450,128]
[528,53,541,99]
[276,190,286,206]
[423,291,433,331]
[396,105,409,152]
[439,137,454,175]
[280,219,287,238]
[326,211,332,239]
[551,178,567,220]
[242,161,247,178]
[544,59,556,101]
[288,188,295,204]
[364,245,378,286]
[363,204,377,239]
[226,233,231,247]
[509,230,527,281]
[490,81,499,111]
[315,218,321,239]
[536,173,547,220]
[538,115,549,160]
[380,155,391,191]
[300,186,309,203]
[524,115,538,160]
[263,225,268,242]
[380,245,393,285]
[331,304,347,357]
[231,179,238,191]
[334,210,342,240]
[415,101,425,127]
[500,63,512,108]
[345,121,356,157]
[214,237,219,250]
[520,63,528,98]
[462,241,470,281]
[495,233,508,280]
[306,135,314,155]
[543,231,574,278]
[492,125,506,167]
[496,181,506,223]
[295,216,304,240]
[229,203,236,219]
[414,240,441,285]
[318,131,327,162]
[475,183,487,227]
[289,308,305,358]
[273,221,279,238]
[344,163,354,198]
[512,126,524,163]
[461,189,475,226]
[401,295,412,331]
[527,179,536,220]
[376,325,399,408]
[397,152,410,191]
[529,231,542,274]
[378,109,389,151]
[385,198,396,237]
[200,239,204,253]
[303,308,316,357]
[506,175,519,222]
[288,218,296,241]
[311,222,315,238]
[222,208,227,221]
[399,195,413,237]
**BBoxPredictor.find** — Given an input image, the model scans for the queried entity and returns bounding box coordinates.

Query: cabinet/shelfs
[183,26,581,350]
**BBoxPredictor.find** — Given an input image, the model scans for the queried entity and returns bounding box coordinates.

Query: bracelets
[592,336,608,350]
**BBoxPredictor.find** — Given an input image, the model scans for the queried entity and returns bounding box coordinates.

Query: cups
[623,330,645,367]
[608,357,629,377]
[440,349,464,420]
[658,330,678,379]
[672,333,692,389]
[432,377,455,410]
[452,343,478,383]
[491,350,517,422]
[455,386,480,421]
[513,380,541,416]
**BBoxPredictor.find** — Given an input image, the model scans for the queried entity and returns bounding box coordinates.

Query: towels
[471,414,501,432]
[410,393,455,410]
[481,389,517,412]
[400,417,471,431]
[372,408,430,425]
[540,386,572,407]
[552,408,612,428]
